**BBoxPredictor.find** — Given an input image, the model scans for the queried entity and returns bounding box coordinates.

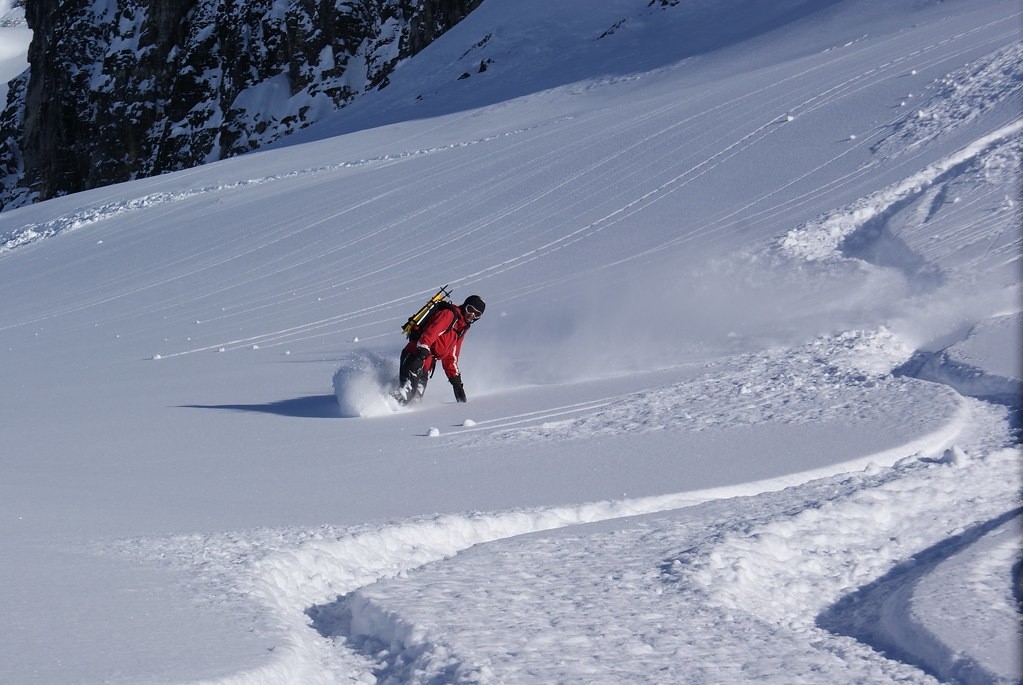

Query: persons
[391,295,486,405]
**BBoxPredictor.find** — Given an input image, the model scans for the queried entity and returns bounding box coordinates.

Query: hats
[465,295,486,314]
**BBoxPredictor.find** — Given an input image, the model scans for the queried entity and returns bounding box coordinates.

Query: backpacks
[402,285,466,341]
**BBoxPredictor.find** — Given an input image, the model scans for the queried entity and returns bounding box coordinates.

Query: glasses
[465,304,482,319]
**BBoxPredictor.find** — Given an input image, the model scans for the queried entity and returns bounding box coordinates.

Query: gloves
[409,347,431,377]
[449,373,467,403]
